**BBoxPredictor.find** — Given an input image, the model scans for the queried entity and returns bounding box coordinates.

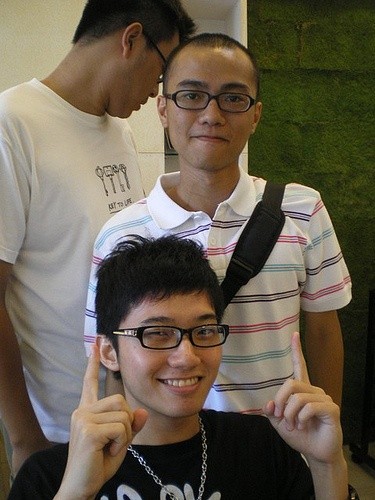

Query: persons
[84,35,353,473]
[0,0,197,488]
[7,232,360,500]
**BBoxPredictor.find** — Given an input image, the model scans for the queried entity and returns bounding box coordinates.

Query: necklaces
[126,414,207,500]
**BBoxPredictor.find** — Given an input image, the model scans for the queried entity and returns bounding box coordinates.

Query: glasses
[142,31,166,84]
[163,89,256,113]
[112,323,230,350]
